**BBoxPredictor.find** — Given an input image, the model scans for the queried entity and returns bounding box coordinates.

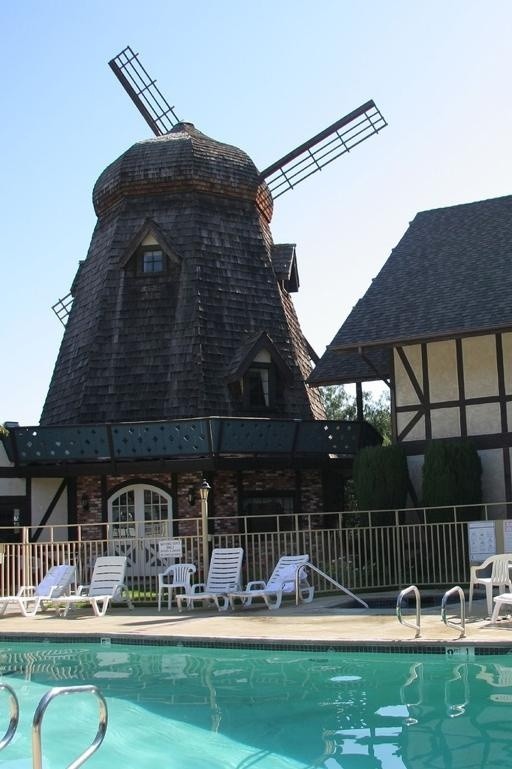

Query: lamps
[198,478,212,500]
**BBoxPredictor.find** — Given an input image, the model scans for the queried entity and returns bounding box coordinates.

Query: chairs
[152,548,316,614]
[1,555,134,623]
[468,553,511,625]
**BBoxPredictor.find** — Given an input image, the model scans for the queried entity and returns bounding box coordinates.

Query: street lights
[198,477,213,610]
